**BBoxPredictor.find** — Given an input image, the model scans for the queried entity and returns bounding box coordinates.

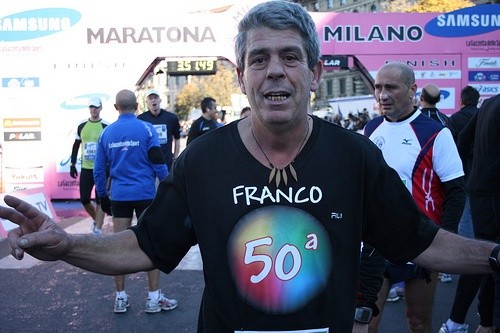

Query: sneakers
[144,289,178,314]
[114,294,130,312]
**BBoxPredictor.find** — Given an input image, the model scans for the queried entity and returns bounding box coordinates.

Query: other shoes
[439,318,469,333]
[392,281,405,296]
[385,285,399,302]
[91,221,102,235]
[441,274,453,283]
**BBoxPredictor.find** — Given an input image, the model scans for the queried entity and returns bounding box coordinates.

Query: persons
[71,63,500,333]
[0,1,499,333]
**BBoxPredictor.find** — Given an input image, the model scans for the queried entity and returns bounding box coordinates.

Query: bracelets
[70,164,76,167]
[488,245,500,272]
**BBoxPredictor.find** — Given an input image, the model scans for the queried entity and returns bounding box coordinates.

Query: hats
[145,89,160,97]
[89,96,101,108]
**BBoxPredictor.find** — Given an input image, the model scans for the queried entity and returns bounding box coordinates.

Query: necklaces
[251,118,311,168]
